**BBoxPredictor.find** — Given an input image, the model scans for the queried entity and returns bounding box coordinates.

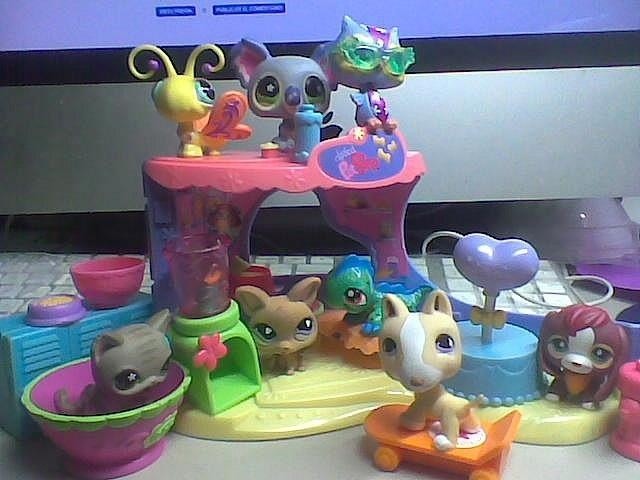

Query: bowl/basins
[20,358,192,476]
[69,257,147,310]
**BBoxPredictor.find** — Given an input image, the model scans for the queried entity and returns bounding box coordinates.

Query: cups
[163,232,231,318]
